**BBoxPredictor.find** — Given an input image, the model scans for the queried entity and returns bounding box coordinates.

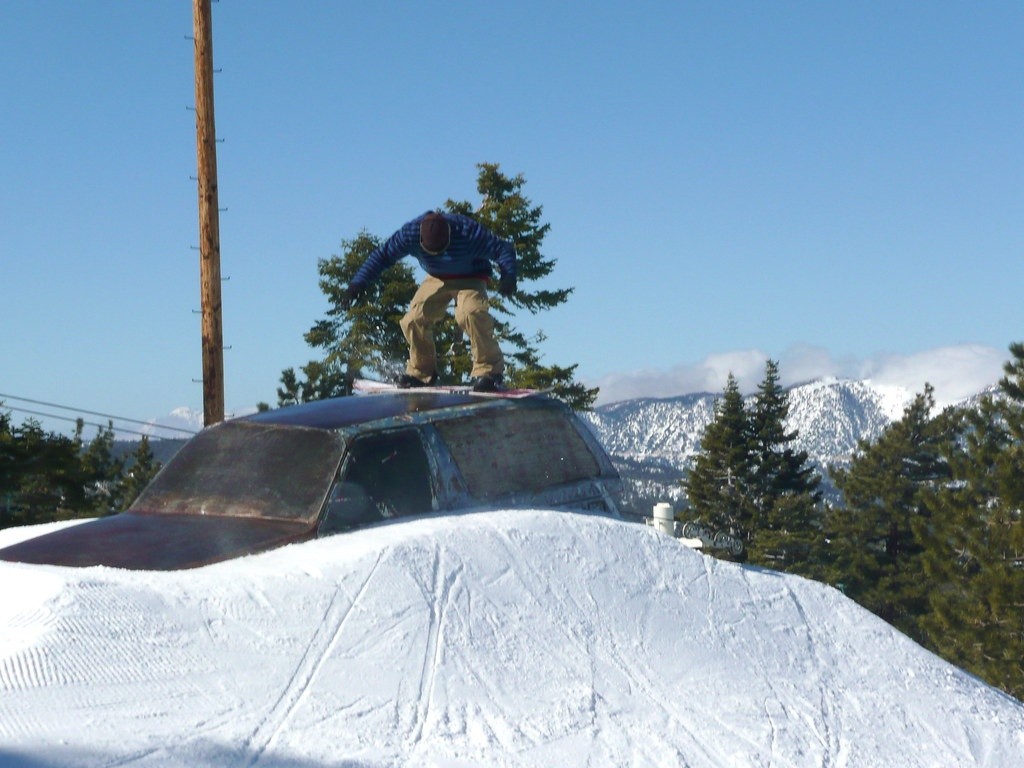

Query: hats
[420,214,451,256]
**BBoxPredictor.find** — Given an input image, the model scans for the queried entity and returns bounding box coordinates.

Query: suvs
[1,373,648,580]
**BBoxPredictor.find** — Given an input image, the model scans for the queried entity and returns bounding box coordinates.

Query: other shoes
[473,374,504,393]
[399,373,437,389]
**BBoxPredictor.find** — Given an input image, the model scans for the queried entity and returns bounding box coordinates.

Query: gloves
[345,282,366,298]
[497,273,516,300]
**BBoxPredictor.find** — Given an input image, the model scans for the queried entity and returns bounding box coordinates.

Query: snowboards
[352,378,556,400]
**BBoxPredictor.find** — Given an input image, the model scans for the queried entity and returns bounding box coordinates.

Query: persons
[347,209,517,392]
